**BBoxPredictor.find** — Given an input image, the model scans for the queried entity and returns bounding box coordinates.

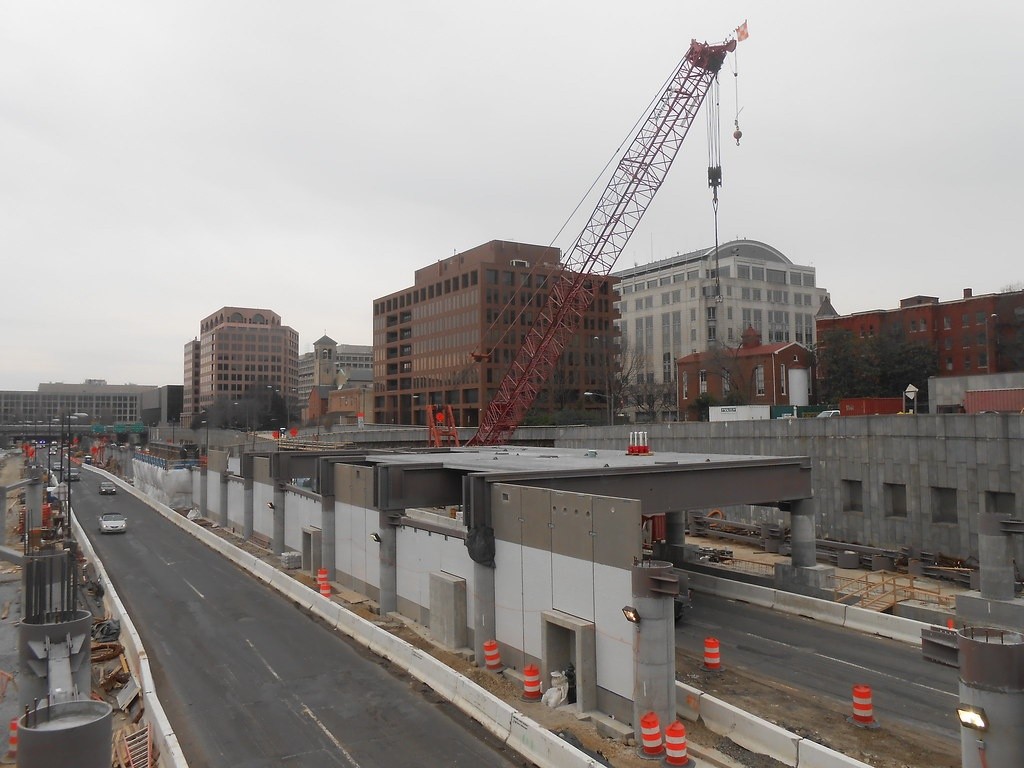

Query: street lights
[985,313,997,374]
[234,400,250,434]
[274,389,297,430]
[201,420,209,458]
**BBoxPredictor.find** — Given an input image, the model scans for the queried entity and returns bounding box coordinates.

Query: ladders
[122,720,153,768]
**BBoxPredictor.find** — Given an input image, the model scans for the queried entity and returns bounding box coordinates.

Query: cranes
[423,39,738,455]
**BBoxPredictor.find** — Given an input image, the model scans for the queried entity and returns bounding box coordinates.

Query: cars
[64,468,82,482]
[36,440,70,455]
[817,410,842,417]
[97,512,128,535]
[98,481,116,495]
[52,462,65,471]
